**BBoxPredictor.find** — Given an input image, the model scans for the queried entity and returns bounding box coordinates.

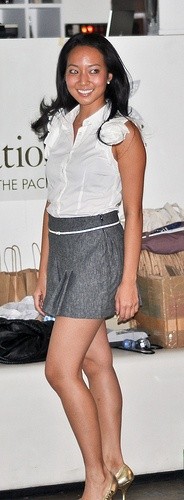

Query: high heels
[78,472,118,500]
[115,464,134,500]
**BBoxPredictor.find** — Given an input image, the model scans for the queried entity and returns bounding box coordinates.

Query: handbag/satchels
[119,202,184,234]
[0,243,41,306]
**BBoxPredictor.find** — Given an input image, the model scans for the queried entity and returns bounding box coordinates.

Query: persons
[30,32,146,500]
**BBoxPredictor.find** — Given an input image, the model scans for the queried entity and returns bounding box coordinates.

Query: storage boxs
[128,249,183,349]
